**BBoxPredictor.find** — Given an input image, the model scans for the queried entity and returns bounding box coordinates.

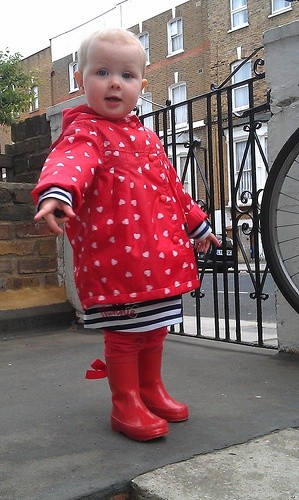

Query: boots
[86,358,169,440]
[137,349,188,423]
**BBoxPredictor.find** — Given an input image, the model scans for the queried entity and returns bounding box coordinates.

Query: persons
[29,28,221,443]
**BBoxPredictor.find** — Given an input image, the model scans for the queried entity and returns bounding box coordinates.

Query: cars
[195,235,234,274]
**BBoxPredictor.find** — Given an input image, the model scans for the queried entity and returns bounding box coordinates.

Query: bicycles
[259,127,299,315]
